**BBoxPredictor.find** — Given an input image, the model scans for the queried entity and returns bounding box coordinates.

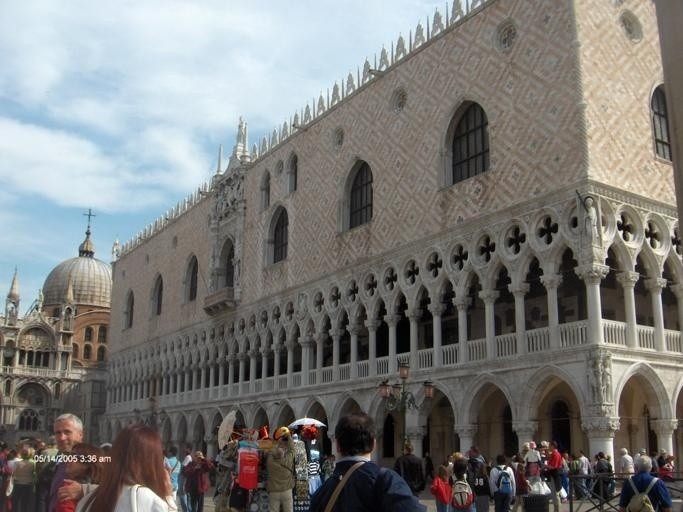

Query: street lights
[132,396,167,431]
[378,358,436,444]
[44,411,54,436]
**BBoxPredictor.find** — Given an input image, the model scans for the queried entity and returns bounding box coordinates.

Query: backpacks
[451,474,473,510]
[626,476,659,512]
[496,466,513,494]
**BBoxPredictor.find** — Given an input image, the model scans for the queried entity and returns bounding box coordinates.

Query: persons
[583,198,598,244]
[8,307,14,325]
[237,116,245,143]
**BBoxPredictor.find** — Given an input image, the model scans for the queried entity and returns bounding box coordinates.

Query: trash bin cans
[521,494,550,512]
